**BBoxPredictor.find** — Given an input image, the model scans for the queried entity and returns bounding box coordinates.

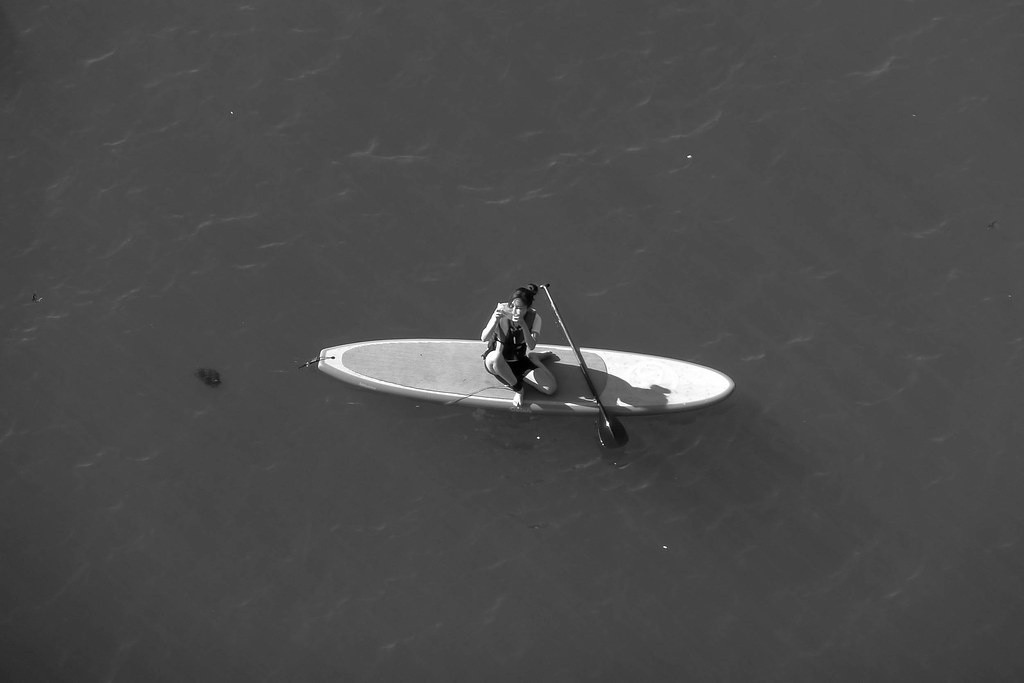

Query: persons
[481,283,558,409]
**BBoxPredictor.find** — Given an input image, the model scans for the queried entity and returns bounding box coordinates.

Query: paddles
[537,280,632,468]
